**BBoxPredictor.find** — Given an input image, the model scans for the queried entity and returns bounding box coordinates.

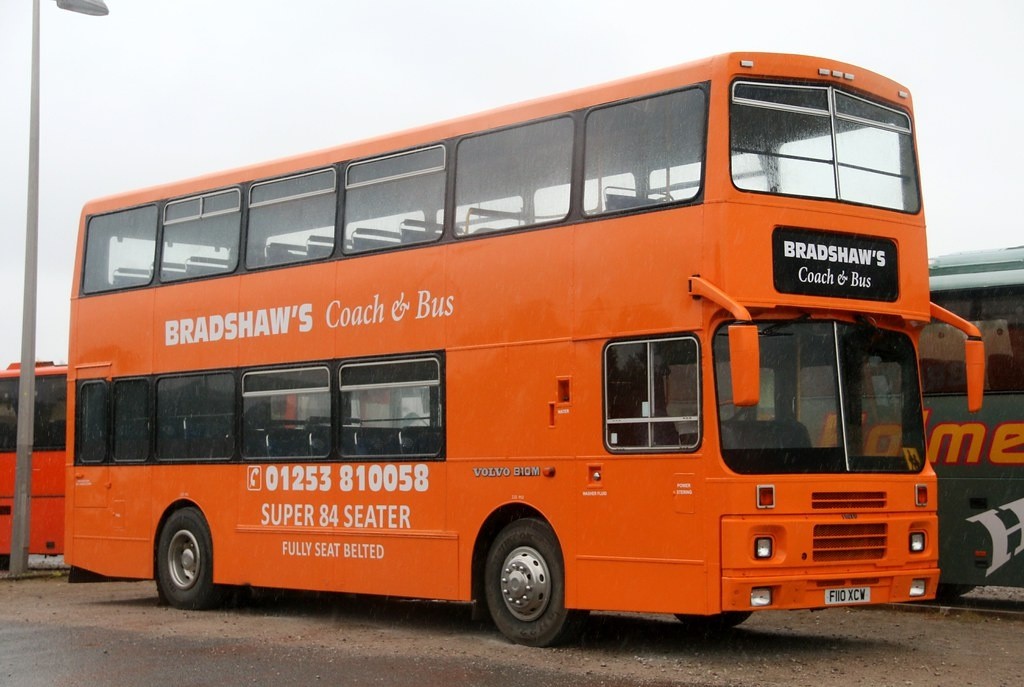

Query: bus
[0,360,390,572]
[61,50,987,649]
[664,245,1024,609]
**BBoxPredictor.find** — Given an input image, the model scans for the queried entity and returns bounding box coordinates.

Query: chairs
[112,187,673,288]
[149,417,441,458]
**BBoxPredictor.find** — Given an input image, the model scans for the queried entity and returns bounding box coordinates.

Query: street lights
[9,0,112,573]
